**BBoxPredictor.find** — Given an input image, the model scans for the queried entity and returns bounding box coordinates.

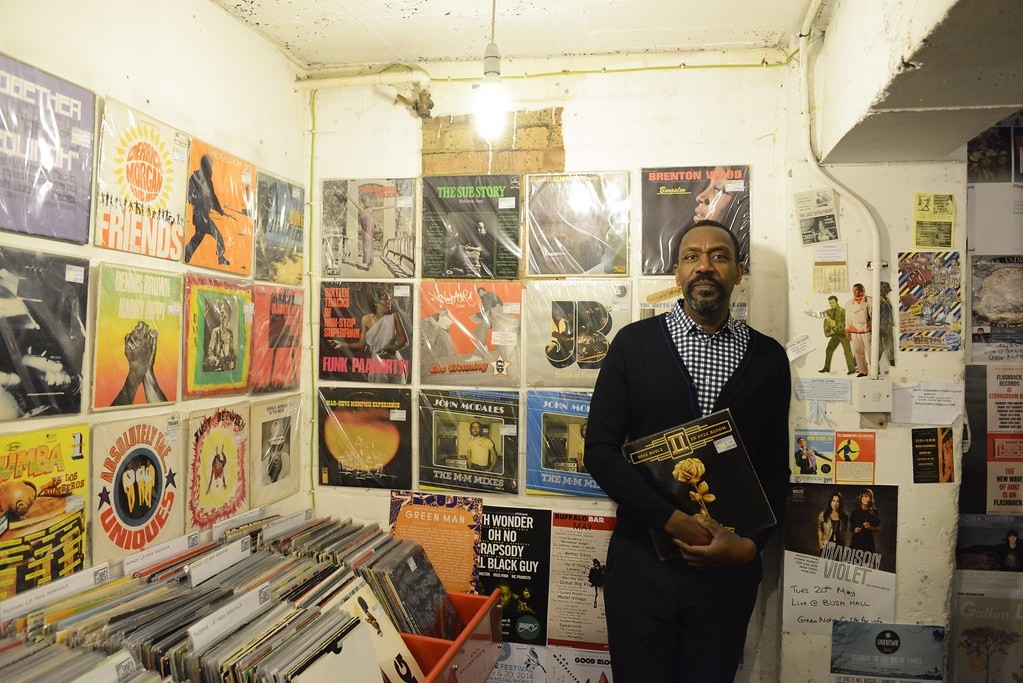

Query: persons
[582,219,793,682]
[90,137,548,643]
[691,165,750,274]
[802,281,896,380]
[795,437,833,476]
[817,220,834,241]
[849,489,882,570]
[529,175,626,275]
[993,529,1021,572]
[835,439,859,461]
[818,491,849,562]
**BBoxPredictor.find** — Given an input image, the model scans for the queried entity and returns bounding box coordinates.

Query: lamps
[469,1,510,145]
